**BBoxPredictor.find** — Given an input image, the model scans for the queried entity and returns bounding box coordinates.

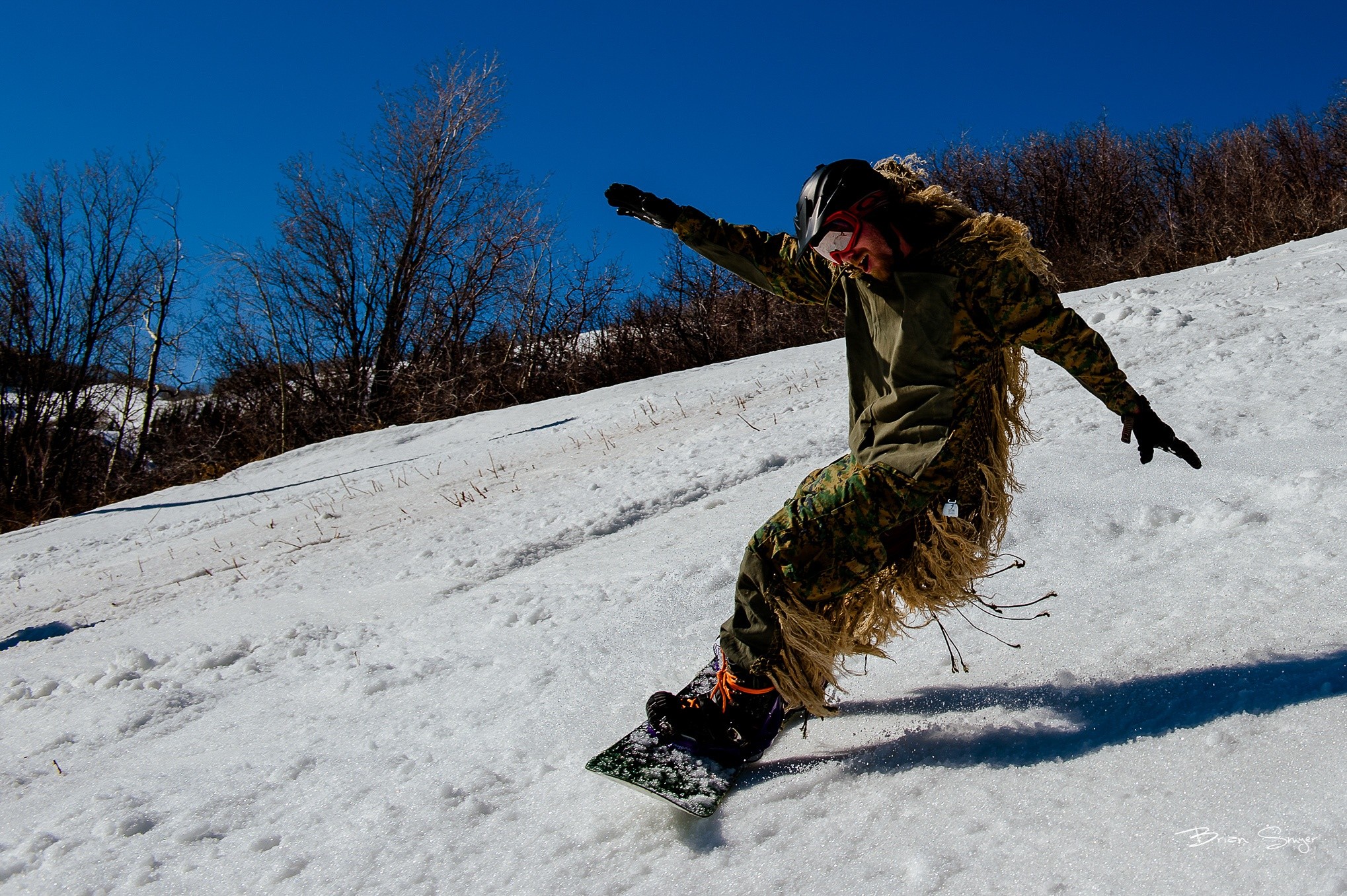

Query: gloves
[604,183,681,230]
[1121,395,1203,469]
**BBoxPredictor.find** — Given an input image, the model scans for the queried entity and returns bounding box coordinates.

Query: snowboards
[585,636,837,822]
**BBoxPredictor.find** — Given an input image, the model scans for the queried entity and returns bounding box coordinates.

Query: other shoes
[646,657,782,764]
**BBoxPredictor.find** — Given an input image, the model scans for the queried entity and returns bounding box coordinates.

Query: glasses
[807,188,890,265]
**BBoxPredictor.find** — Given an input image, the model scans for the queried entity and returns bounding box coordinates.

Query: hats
[794,158,893,266]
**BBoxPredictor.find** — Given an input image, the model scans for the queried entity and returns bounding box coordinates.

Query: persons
[604,153,1202,766]
